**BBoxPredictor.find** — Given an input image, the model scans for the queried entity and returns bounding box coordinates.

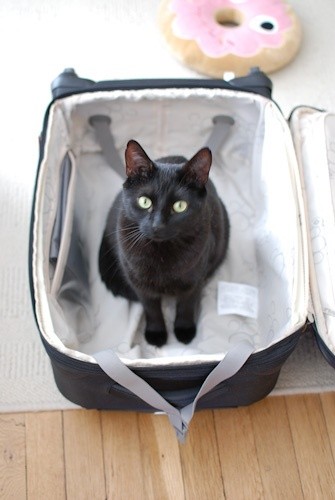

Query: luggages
[28,65,334,444]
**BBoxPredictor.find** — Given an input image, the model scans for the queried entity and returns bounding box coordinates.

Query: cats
[97,139,231,347]
